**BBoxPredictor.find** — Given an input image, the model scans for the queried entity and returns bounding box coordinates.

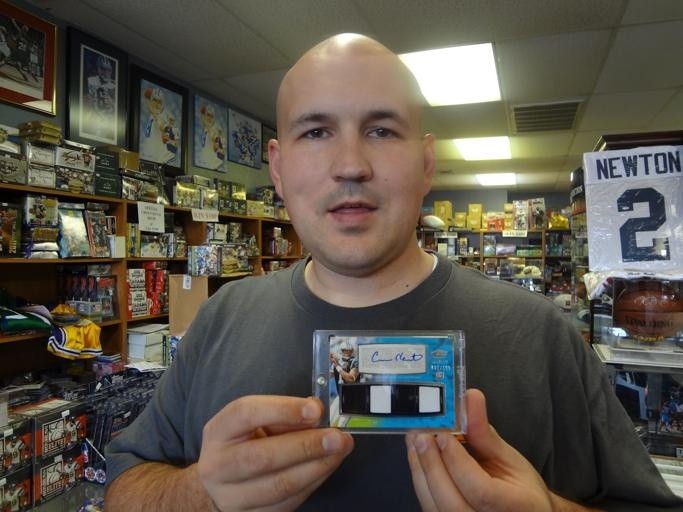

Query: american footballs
[331,354,338,363]
[423,215,445,228]
[554,294,583,311]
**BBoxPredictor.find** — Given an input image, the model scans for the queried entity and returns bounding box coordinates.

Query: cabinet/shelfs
[418,228,571,297]
[0,183,302,368]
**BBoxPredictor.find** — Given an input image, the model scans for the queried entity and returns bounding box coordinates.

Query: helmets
[204,104,215,121]
[96,55,113,75]
[149,88,165,110]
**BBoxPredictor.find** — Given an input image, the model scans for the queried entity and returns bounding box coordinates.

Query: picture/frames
[0,9,190,177]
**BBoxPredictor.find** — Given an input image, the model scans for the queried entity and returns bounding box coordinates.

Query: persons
[145,88,179,164]
[0,18,41,76]
[328,341,358,384]
[104,33,683,512]
[200,104,227,170]
[89,57,115,114]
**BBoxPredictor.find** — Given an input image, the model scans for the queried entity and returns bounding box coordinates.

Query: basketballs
[613,280,682,341]
